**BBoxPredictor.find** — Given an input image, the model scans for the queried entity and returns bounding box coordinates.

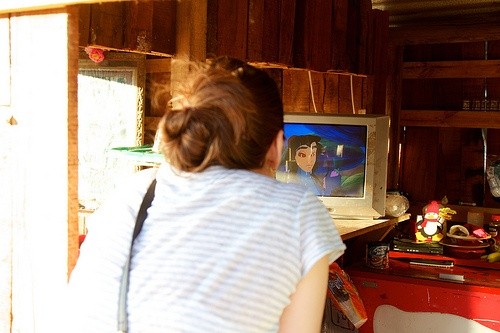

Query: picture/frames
[78,58,144,212]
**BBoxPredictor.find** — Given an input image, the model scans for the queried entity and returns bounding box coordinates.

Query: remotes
[409,258,454,268]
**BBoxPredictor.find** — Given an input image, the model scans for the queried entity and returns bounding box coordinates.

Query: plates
[447,234,491,240]
[439,238,496,248]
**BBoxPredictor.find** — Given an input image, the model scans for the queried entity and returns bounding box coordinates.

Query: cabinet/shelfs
[322,0,500,333]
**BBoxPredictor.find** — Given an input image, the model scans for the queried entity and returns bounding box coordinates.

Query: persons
[61,56,346,333]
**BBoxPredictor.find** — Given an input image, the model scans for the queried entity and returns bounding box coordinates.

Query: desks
[330,212,411,239]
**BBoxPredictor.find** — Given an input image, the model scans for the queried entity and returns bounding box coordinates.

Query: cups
[364,241,390,270]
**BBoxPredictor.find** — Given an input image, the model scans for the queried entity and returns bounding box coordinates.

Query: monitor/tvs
[276,112,390,221]
